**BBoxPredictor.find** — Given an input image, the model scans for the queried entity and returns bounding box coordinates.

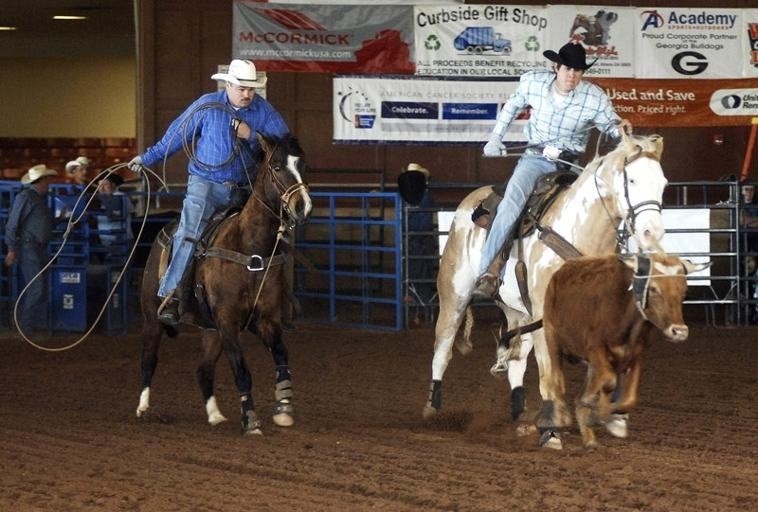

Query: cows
[542,251,716,454]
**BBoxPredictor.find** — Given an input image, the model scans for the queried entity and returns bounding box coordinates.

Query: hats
[94,169,123,185]
[21,164,57,185]
[543,44,598,69]
[211,59,268,88]
[65,156,92,172]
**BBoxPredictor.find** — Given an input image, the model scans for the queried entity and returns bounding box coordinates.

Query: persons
[128,59,291,325]
[471,44,633,303]
[64,157,132,245]
[3,164,57,335]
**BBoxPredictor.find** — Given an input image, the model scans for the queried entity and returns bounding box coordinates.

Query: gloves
[128,155,144,172]
[484,134,507,157]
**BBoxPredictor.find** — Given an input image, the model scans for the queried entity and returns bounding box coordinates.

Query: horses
[132,130,315,437]
[421,122,670,439]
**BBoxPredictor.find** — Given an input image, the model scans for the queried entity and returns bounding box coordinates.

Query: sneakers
[159,301,179,322]
[473,276,500,301]
